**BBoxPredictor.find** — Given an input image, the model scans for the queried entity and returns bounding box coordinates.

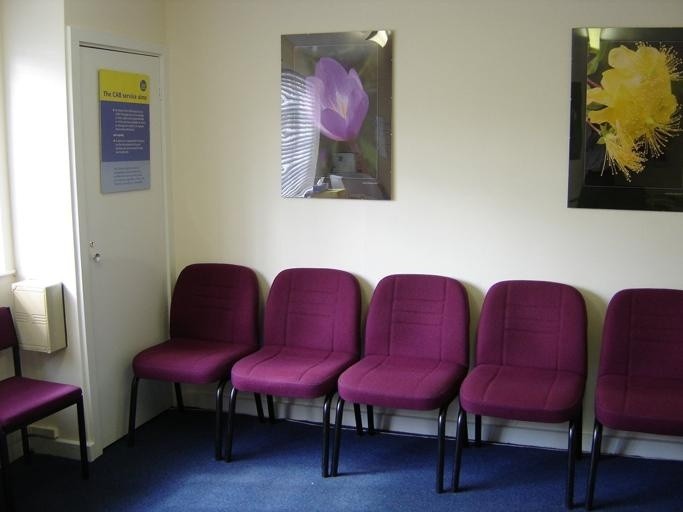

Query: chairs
[224,268,360,478]
[129,263,264,462]
[449,280,588,511]
[330,274,468,492]
[585,290,682,512]
[0,306,88,497]
